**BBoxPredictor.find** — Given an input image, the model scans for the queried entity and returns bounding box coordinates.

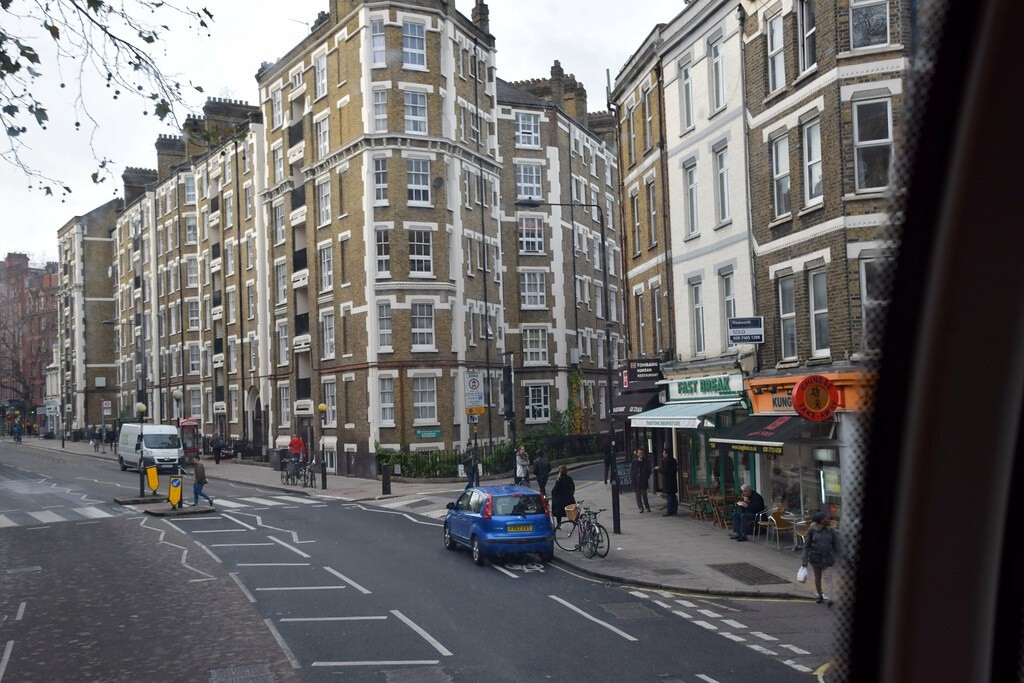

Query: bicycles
[280,458,317,488]
[554,499,611,560]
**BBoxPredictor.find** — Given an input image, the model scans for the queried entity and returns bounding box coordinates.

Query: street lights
[317,403,330,490]
[497,350,517,485]
[513,198,623,535]
[135,403,147,497]
[171,389,184,434]
[101,319,146,412]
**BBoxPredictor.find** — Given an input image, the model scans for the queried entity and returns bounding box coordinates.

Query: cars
[444,483,556,566]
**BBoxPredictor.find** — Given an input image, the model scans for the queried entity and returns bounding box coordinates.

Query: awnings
[628,402,739,428]
[708,416,811,456]
[608,391,658,415]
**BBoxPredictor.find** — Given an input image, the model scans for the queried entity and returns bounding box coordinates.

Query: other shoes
[640,509,643,513]
[731,533,740,539]
[647,507,650,512]
[662,511,676,517]
[737,536,747,541]
[209,497,213,506]
[191,504,198,506]
[816,596,822,603]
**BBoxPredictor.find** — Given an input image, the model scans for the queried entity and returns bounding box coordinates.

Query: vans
[116,422,185,475]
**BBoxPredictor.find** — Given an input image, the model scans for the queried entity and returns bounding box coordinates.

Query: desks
[780,515,810,551]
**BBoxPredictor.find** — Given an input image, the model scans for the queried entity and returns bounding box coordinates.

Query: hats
[813,511,828,523]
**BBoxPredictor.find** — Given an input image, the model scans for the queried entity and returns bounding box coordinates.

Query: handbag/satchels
[797,567,808,583]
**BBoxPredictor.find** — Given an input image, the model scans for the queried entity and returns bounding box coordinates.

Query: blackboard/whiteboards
[616,461,632,491]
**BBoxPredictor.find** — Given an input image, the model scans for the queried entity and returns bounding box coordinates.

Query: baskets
[565,504,577,520]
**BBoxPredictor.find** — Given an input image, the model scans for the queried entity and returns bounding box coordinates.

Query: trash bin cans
[273,448,288,471]
[71,430,80,442]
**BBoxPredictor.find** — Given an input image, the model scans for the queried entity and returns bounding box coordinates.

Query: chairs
[686,484,740,530]
[753,507,778,544]
[767,510,797,548]
[792,520,818,553]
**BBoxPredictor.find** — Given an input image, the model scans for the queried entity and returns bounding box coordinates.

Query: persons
[92,428,102,452]
[802,512,838,603]
[630,449,651,513]
[655,448,678,517]
[532,449,551,497]
[210,433,221,464]
[729,484,768,542]
[14,423,21,432]
[551,465,576,528]
[461,443,476,490]
[27,423,31,436]
[190,456,213,507]
[602,444,618,485]
[515,445,530,485]
[288,434,306,462]
